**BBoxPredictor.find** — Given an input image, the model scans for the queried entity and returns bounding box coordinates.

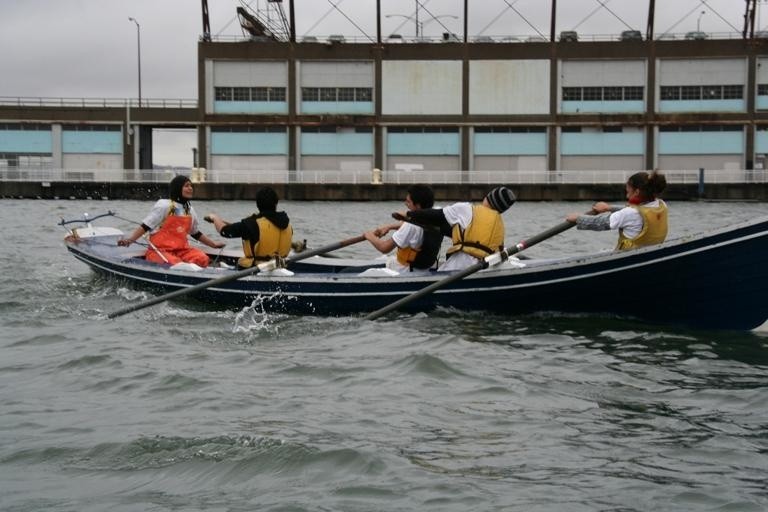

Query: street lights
[128,17,143,107]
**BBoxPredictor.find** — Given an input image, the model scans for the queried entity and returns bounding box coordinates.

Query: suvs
[620,29,642,39]
[562,30,577,40]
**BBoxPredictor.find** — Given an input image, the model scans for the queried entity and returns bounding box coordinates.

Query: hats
[487,185,517,213]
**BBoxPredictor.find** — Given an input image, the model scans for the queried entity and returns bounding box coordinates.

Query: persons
[207,188,293,271]
[398,187,526,271]
[566,172,669,253]
[117,174,226,267]
[363,185,443,274]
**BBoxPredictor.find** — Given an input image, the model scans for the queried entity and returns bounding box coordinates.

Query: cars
[687,30,704,38]
[326,35,341,41]
[387,34,401,41]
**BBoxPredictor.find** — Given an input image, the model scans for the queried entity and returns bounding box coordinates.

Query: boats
[57,187,762,332]
[62,213,767,329]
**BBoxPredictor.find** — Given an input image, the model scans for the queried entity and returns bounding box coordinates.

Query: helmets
[168,176,187,193]
